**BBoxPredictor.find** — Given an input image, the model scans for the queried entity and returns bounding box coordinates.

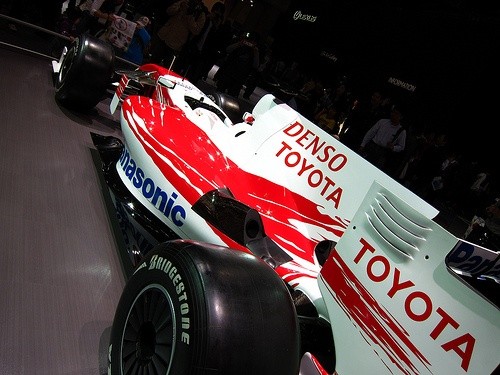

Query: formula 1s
[51,35,499,374]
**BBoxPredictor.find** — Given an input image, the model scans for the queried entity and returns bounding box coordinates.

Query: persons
[175,2,226,82]
[49,1,153,72]
[359,106,407,169]
[315,105,338,135]
[157,0,206,68]
